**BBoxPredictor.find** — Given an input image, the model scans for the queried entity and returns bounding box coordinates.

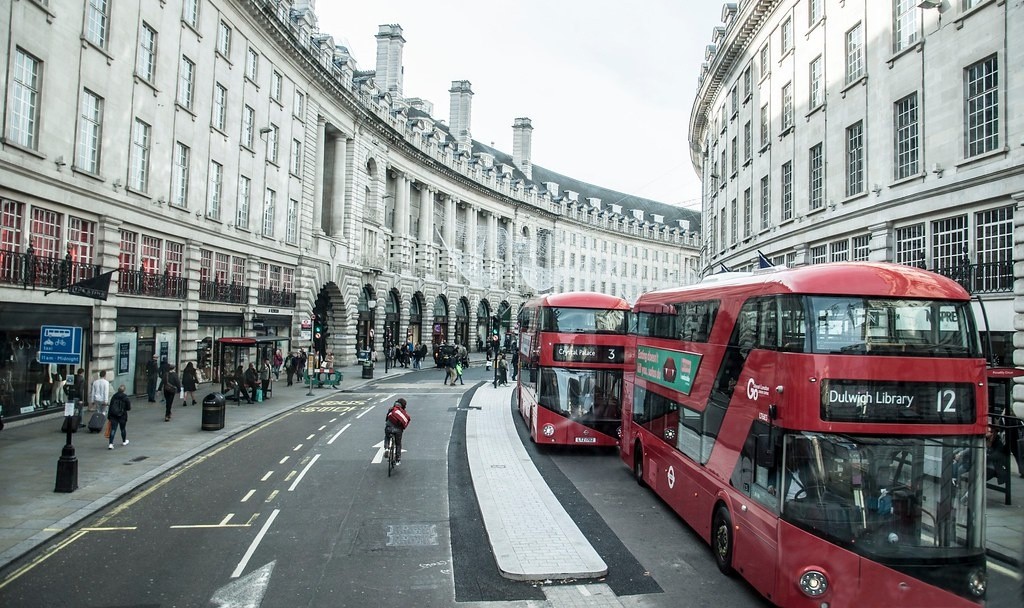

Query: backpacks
[63,375,76,395]
[286,357,294,368]
[388,405,410,429]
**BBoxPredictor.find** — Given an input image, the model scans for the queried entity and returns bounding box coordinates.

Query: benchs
[225,375,262,390]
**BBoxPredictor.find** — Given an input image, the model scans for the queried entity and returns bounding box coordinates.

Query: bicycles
[385,422,405,478]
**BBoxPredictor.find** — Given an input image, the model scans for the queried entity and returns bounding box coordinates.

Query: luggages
[88,404,106,433]
[61,403,83,432]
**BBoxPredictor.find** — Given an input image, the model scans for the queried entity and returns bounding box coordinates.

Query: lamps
[917,1,942,11]
[260,125,272,138]
[710,173,720,180]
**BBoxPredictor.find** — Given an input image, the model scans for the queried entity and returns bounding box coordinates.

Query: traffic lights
[314,312,323,340]
[493,314,499,346]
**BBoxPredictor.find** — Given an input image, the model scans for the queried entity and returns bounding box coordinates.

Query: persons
[92,369,109,415]
[30,350,66,409]
[145,354,161,403]
[259,359,273,401]
[107,384,131,449]
[951,409,1024,506]
[433,338,468,386]
[272,349,335,387]
[243,362,259,402]
[384,398,407,467]
[158,357,176,402]
[158,365,182,421]
[63,368,87,429]
[477,336,519,387]
[387,339,428,370]
[233,365,254,404]
[370,349,378,370]
[182,362,199,406]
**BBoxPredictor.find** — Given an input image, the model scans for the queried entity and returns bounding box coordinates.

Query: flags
[759,250,774,268]
[720,262,730,272]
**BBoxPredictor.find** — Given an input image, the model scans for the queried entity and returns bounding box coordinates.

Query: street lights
[367,297,377,362]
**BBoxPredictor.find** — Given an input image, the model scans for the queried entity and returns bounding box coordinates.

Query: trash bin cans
[202,392,225,430]
[362,360,374,379]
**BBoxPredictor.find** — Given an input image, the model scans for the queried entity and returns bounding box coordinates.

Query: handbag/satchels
[255,389,262,402]
[165,383,176,393]
[104,421,111,438]
[486,361,492,367]
[180,387,185,398]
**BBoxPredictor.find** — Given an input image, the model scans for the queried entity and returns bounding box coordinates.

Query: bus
[514,290,631,451]
[618,259,1024,608]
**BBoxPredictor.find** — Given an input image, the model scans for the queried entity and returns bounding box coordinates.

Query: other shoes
[385,449,388,458]
[396,461,401,465]
[122,440,129,446]
[183,401,186,406]
[248,401,254,404]
[109,444,114,449]
[165,415,170,421]
[193,400,196,405]
[149,399,155,402]
